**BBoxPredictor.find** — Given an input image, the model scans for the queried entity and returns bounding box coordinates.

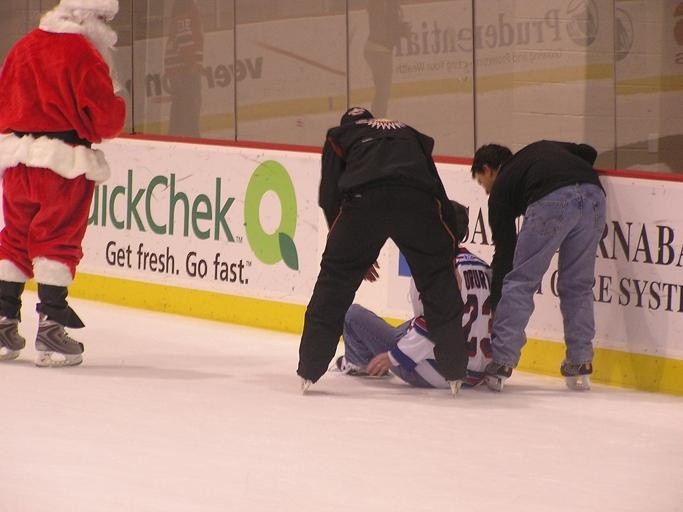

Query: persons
[363,0,413,118]
[297,107,468,400]
[0,0,127,355]
[336,201,493,389]
[164,0,205,139]
[470,141,608,393]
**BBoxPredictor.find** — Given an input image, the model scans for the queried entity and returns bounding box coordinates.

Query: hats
[340,107,375,127]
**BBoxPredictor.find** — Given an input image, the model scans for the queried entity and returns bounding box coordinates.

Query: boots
[334,355,392,378]
[0,315,26,351]
[559,360,595,378]
[442,358,467,381]
[482,362,516,380]
[297,361,328,383]
[32,313,84,355]
